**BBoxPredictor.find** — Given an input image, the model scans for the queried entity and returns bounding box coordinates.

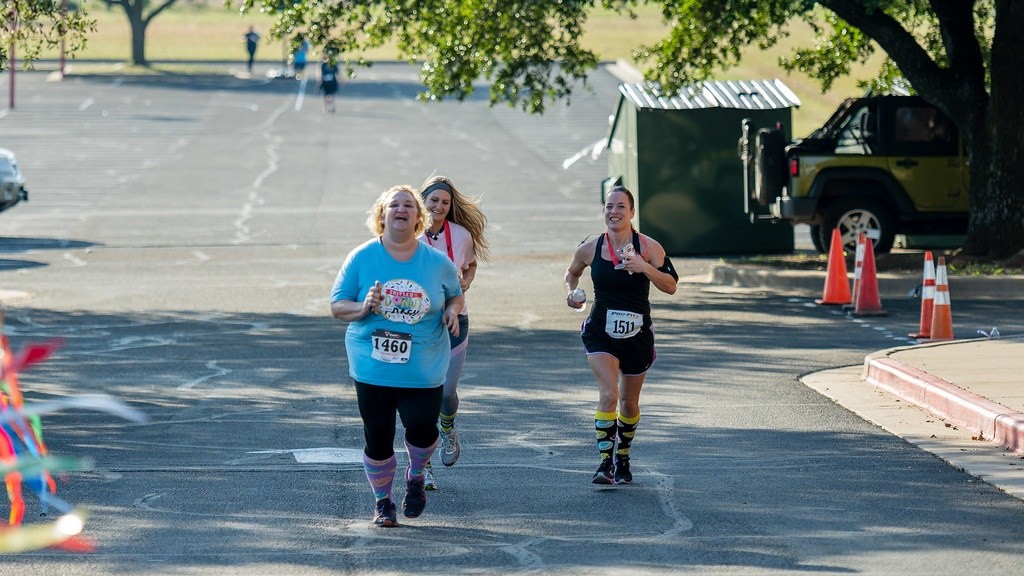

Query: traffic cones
[814,229,851,305]
[843,233,889,317]
[906,250,954,343]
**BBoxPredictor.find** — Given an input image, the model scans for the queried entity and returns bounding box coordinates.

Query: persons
[330,185,465,528]
[316,62,342,112]
[240,23,259,73]
[563,186,679,484]
[292,33,310,81]
[420,175,493,491]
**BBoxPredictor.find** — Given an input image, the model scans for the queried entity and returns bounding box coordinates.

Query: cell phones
[622,243,636,275]
[371,281,381,316]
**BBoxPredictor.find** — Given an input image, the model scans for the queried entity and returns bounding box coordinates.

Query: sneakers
[373,497,399,527]
[424,464,437,490]
[439,419,460,466]
[402,466,426,518]
[615,453,632,484]
[592,458,615,483]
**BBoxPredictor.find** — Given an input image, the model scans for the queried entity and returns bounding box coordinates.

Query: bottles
[573,288,586,312]
[990,326,1000,338]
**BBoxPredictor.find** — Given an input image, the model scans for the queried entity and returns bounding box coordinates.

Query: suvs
[740,93,971,257]
